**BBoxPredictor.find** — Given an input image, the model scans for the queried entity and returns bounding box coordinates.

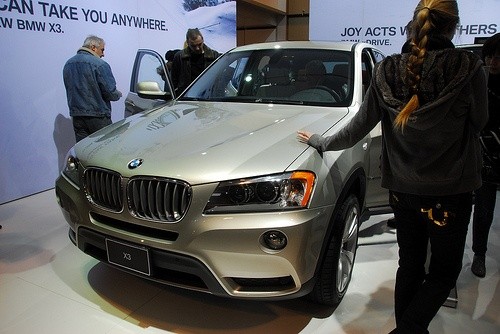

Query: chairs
[254,53,367,97]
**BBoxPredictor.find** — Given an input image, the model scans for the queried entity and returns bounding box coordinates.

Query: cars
[54,41,389,308]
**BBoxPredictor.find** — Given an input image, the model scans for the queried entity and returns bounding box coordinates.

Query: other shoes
[471,255,486,277]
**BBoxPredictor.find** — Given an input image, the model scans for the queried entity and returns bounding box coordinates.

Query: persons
[63,36,123,143]
[156,28,235,101]
[297,0,500,334]
